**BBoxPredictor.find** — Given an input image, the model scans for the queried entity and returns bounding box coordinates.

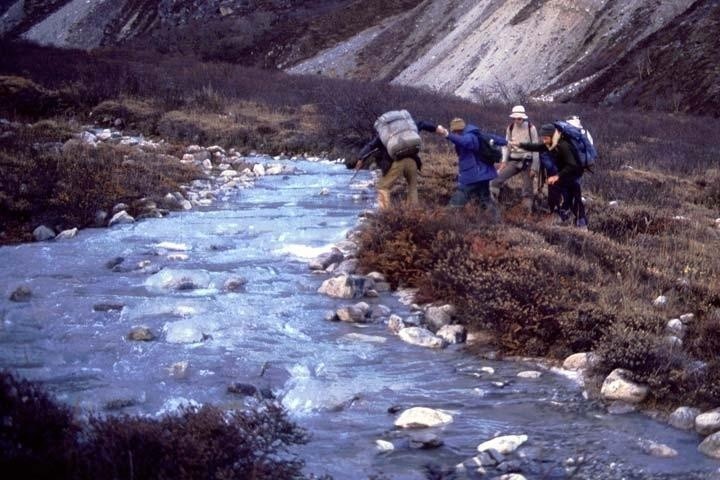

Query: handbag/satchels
[555,115,598,174]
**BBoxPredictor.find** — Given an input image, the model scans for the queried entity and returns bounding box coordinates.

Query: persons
[511,115,598,231]
[535,147,567,213]
[440,118,514,210]
[489,105,540,211]
[356,110,444,210]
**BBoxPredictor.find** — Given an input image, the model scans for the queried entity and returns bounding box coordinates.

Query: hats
[450,117,465,133]
[509,104,528,120]
[539,123,555,137]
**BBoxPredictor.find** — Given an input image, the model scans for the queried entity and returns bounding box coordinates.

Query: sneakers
[471,129,502,165]
[554,206,571,222]
[575,218,589,232]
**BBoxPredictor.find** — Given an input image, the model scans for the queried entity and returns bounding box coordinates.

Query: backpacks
[373,108,422,157]
